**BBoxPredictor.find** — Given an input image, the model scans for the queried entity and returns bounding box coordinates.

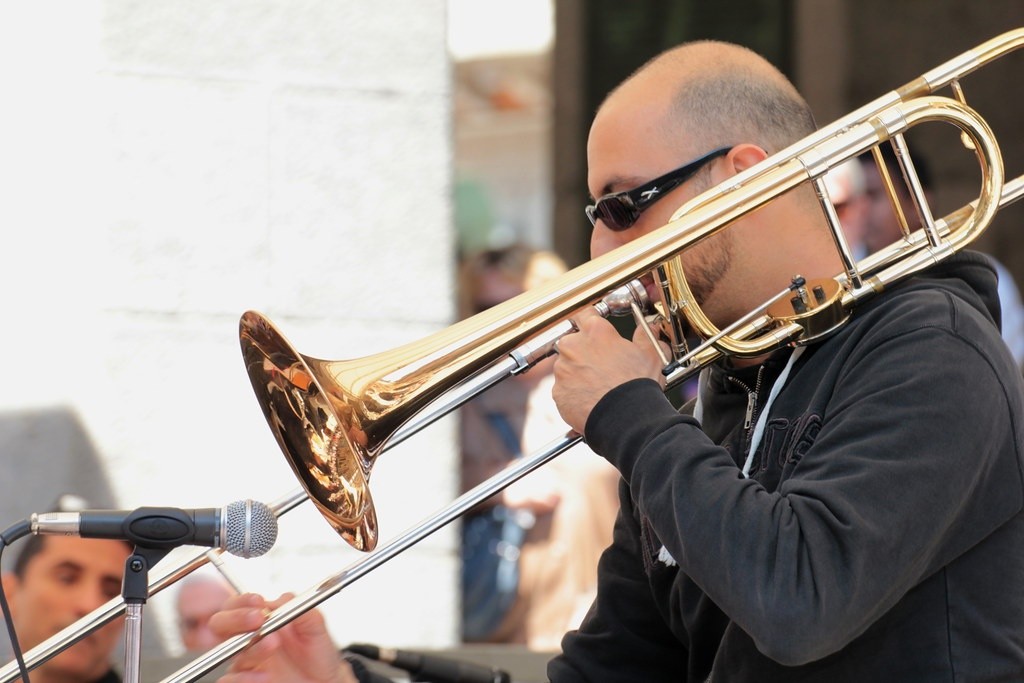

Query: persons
[150,575,234,683]
[209,40,1024,683]
[0,493,135,682]
[456,123,1022,653]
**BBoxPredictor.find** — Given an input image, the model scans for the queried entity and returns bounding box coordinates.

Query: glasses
[584,145,734,231]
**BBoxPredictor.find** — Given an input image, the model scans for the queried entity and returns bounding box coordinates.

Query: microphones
[350,640,511,683]
[0,502,283,561]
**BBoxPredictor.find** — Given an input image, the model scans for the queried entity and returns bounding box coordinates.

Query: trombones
[0,25,1023,683]
